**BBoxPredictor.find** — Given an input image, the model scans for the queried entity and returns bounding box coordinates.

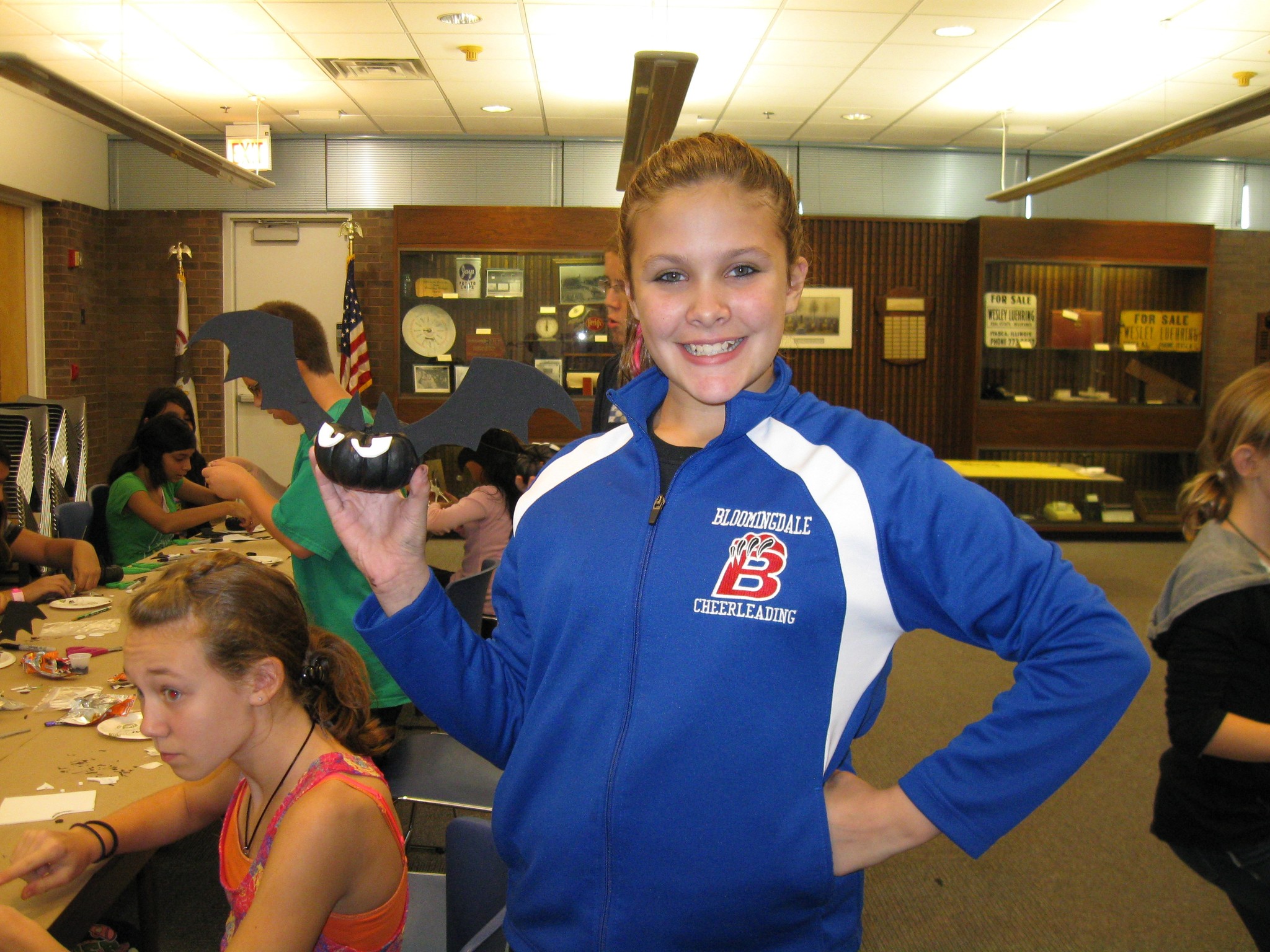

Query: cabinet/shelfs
[391,203,638,447]
[962,211,1217,540]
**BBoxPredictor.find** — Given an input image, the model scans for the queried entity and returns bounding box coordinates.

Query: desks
[0,514,298,952]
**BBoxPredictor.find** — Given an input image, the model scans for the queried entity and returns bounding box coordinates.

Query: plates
[0,651,16,669]
[49,596,112,609]
[247,556,283,564]
[223,524,266,533]
[97,712,153,739]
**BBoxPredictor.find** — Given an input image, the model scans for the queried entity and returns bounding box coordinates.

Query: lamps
[0,46,278,196]
[612,45,701,198]
[982,59,1270,205]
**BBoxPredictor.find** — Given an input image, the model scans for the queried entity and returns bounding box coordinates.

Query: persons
[0,551,411,952]
[309,132,1152,951]
[1145,364,1270,951]
[0,439,101,613]
[105,411,260,567]
[428,430,523,615]
[201,302,410,769]
[109,387,212,539]
[515,246,628,493]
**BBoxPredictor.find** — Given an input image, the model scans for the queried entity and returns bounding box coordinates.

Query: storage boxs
[484,267,524,297]
[566,368,601,393]
[1050,306,1105,349]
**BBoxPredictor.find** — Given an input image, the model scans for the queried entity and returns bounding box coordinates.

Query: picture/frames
[778,286,854,351]
[411,362,452,396]
[550,257,607,306]
[533,356,564,387]
[453,363,470,392]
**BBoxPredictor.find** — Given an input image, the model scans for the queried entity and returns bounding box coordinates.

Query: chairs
[369,551,546,952]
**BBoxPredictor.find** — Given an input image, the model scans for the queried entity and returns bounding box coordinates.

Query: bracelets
[85,820,118,860]
[10,588,25,602]
[69,822,105,862]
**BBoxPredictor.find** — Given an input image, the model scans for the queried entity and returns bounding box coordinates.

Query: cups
[68,653,92,675]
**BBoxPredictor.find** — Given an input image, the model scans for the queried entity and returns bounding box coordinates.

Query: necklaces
[241,707,316,857]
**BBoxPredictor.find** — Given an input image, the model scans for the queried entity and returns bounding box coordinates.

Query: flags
[340,255,372,396]
[175,275,201,454]
